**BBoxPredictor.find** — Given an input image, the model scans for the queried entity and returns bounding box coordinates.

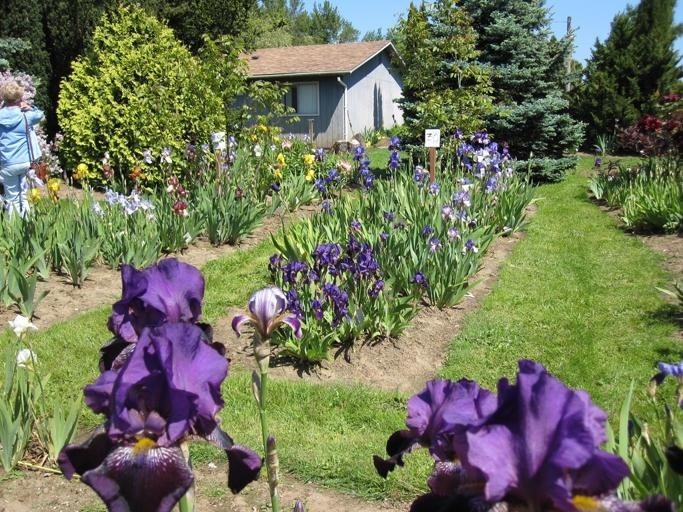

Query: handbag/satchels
[31,162,47,184]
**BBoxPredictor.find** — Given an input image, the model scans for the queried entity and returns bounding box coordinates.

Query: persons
[0,81,44,222]
